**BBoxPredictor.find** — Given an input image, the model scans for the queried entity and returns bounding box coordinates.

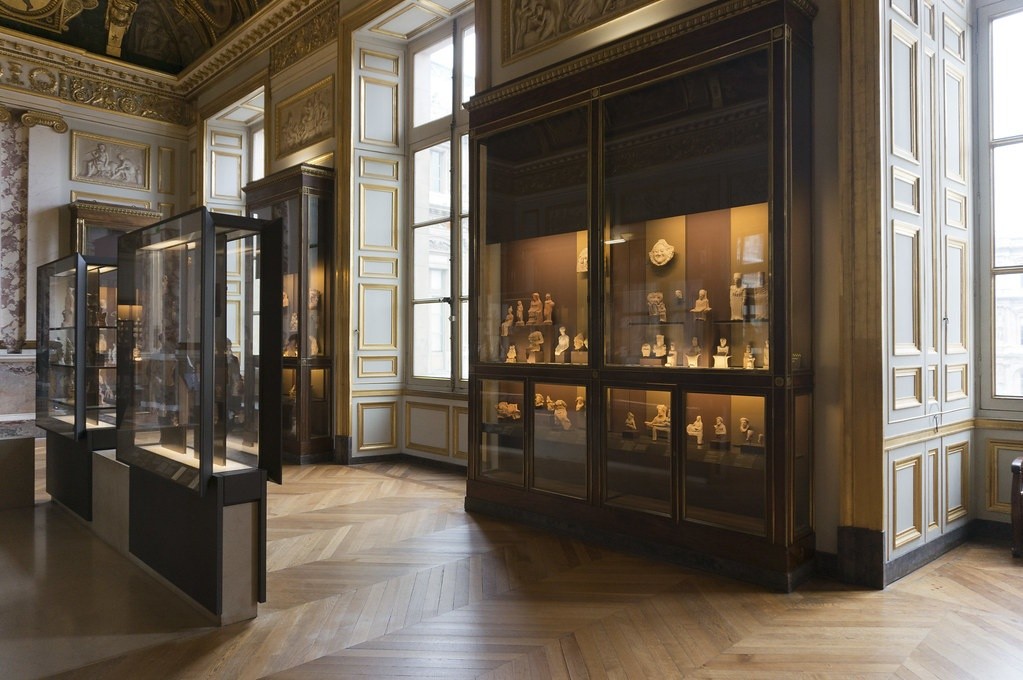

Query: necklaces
[86,144,134,183]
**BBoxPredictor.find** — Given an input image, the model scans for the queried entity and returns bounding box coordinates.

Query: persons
[553,326,588,356]
[575,396,586,413]
[693,415,704,449]
[740,417,749,432]
[501,292,554,336]
[554,399,572,431]
[642,271,769,369]
[644,405,668,428]
[59,286,77,401]
[624,411,637,429]
[713,417,726,437]
[147,325,195,440]
[527,325,544,352]
[215,338,242,433]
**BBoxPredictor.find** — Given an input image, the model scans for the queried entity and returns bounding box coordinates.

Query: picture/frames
[69,129,151,193]
[71,190,152,211]
